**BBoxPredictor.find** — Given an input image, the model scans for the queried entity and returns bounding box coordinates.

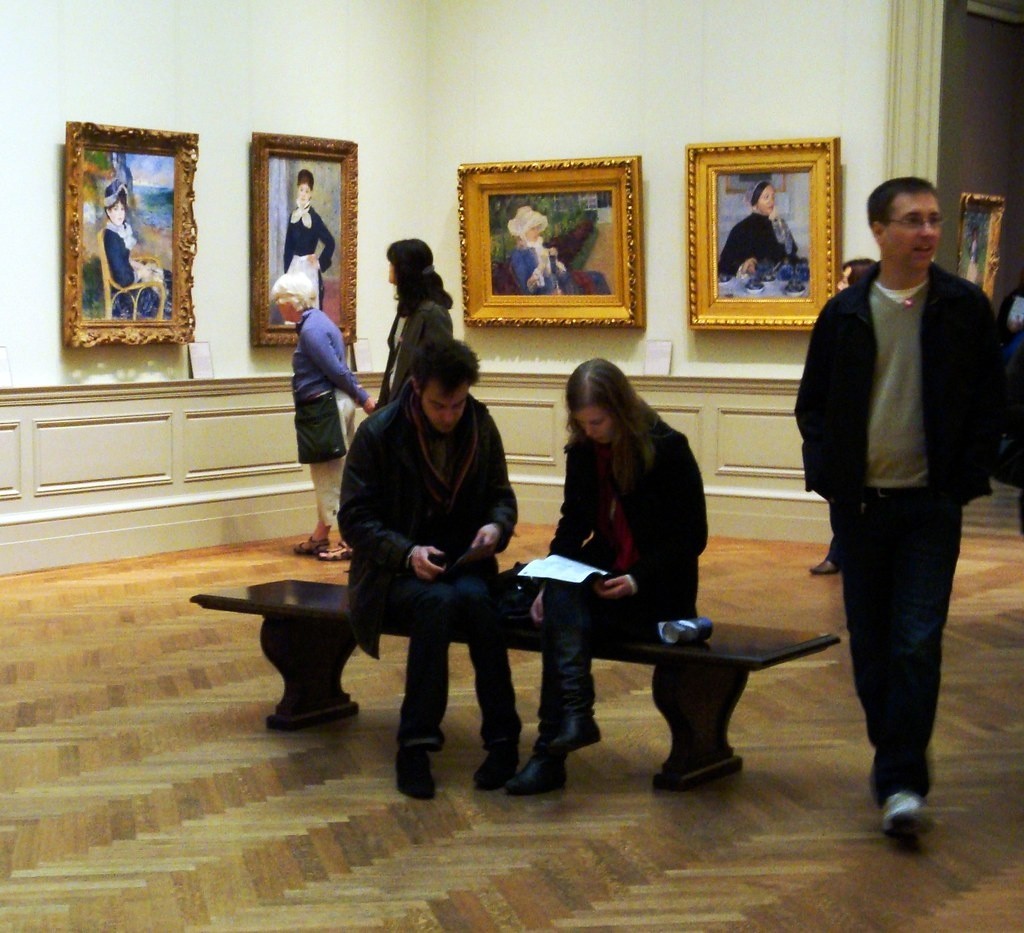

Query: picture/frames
[954,192,1007,302]
[61,120,202,343]
[685,135,842,333]
[250,130,359,347]
[457,156,646,330]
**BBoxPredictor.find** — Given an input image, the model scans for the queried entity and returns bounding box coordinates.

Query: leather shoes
[809,560,840,574]
[551,714,602,753]
[505,756,567,794]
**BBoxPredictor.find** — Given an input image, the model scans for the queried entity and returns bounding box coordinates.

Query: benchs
[189,579,841,793]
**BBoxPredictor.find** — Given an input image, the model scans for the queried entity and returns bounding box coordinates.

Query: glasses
[886,212,948,234]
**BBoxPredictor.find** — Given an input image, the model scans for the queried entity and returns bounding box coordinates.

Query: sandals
[318,543,353,561]
[292,536,330,555]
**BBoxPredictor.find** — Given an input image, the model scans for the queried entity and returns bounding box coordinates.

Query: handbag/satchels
[294,394,347,464]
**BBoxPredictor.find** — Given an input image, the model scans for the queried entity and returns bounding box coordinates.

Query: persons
[810,258,878,573]
[374,238,453,413]
[505,357,708,794]
[336,340,522,799]
[997,281,1024,534]
[272,273,376,560]
[796,177,1008,830]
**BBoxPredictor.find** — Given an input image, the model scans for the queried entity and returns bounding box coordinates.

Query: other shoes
[884,792,929,838]
[474,747,520,789]
[397,748,435,799]
